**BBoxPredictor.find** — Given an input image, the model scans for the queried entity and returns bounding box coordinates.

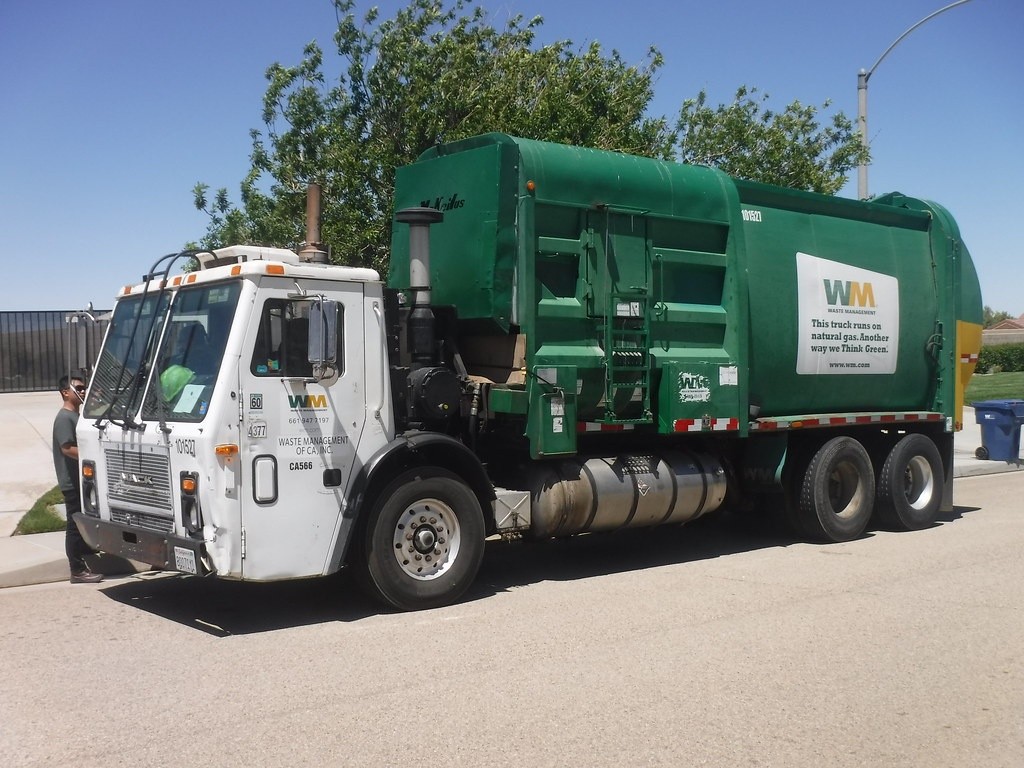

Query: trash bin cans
[973,399,1024,461]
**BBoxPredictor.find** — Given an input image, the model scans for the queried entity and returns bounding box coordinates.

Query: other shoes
[70,571,104,583]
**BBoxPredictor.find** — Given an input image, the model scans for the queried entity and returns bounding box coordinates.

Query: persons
[53,375,103,583]
[164,325,218,375]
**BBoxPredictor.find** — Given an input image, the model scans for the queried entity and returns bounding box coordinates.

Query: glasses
[74,384,86,391]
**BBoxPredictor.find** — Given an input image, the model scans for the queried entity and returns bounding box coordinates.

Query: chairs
[268,318,328,377]
[163,323,222,377]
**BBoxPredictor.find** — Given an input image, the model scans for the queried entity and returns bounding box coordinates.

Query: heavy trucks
[65,131,986,612]
